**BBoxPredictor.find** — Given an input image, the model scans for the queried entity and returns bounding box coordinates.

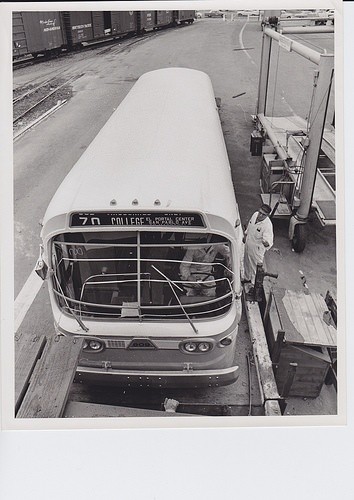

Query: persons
[178,237,233,298]
[240,204,274,289]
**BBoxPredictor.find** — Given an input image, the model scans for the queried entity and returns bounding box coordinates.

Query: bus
[30,65,244,392]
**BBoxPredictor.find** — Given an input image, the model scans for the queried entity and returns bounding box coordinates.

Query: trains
[12,11,196,62]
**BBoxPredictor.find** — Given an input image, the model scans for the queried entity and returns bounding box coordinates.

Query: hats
[259,204,271,215]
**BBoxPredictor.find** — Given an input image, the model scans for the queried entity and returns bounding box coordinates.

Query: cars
[195,10,334,22]
